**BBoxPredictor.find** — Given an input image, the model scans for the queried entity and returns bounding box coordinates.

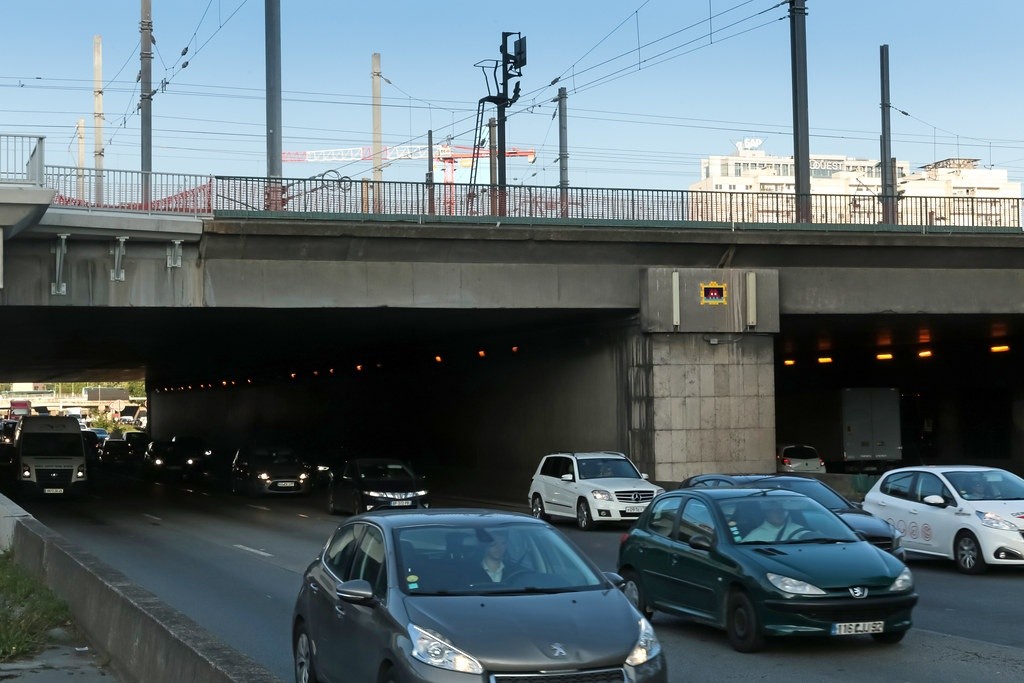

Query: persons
[461,527,523,590]
[598,463,617,476]
[970,478,993,499]
[742,501,823,541]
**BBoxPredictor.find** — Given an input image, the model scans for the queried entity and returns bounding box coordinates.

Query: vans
[13,415,88,501]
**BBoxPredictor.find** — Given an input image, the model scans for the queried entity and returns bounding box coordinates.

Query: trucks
[776,387,902,474]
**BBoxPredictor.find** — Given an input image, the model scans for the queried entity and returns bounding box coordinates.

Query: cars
[616,487,919,652]
[79,424,222,484]
[775,444,827,475]
[318,459,430,517]
[291,507,669,683]
[232,446,314,496]
[862,464,1024,574]
[0,418,20,472]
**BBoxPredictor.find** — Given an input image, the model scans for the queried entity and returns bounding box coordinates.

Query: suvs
[528,451,667,530]
[679,473,906,563]
